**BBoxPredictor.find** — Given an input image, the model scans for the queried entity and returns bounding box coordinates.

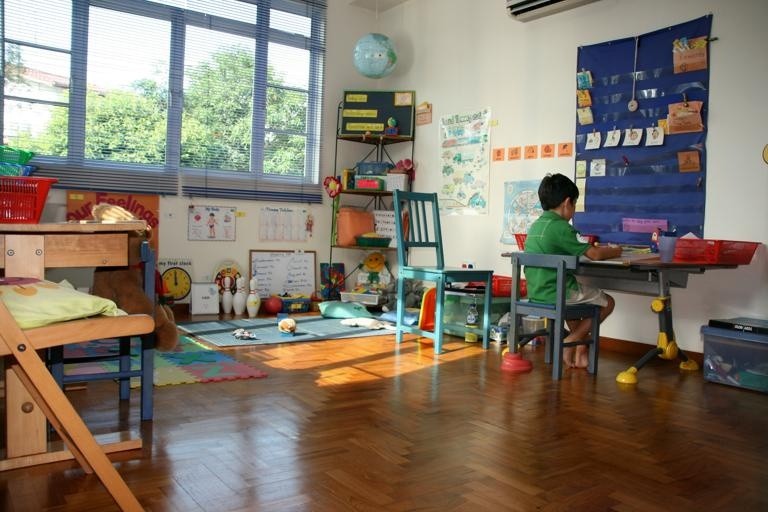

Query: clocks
[158,267,191,301]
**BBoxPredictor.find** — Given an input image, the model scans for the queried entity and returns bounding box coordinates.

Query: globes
[352,32,398,79]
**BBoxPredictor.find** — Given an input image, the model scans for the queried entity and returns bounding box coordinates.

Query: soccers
[264,297,282,313]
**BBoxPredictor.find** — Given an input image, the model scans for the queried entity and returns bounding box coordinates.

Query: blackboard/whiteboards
[249,249,317,300]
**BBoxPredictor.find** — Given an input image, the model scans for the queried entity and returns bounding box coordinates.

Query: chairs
[506,252,600,384]
[387,187,492,357]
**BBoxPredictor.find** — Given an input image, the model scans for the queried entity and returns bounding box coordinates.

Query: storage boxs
[352,173,409,191]
[440,296,510,341]
[354,159,389,175]
[701,318,768,394]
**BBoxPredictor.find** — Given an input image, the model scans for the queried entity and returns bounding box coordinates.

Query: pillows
[315,299,371,320]
[1,277,121,332]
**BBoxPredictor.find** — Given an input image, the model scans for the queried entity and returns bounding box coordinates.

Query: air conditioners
[504,0,598,23]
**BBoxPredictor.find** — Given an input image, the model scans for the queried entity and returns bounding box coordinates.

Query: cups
[658,231,676,262]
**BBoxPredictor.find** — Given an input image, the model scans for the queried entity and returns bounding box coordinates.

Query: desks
[503,247,737,395]
[0,213,152,473]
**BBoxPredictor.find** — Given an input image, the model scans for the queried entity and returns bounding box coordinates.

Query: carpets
[178,315,407,352]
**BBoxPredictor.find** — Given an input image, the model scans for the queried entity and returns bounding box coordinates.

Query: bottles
[465,303,481,344]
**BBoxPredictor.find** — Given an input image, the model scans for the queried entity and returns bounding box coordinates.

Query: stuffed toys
[354,251,392,294]
[89,201,178,350]
[381,278,424,315]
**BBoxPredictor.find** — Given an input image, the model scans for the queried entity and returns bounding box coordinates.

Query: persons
[205,213,217,236]
[522,172,622,367]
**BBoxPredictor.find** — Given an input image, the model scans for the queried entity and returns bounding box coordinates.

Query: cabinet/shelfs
[323,96,415,309]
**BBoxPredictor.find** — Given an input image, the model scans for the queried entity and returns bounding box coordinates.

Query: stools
[0,269,155,511]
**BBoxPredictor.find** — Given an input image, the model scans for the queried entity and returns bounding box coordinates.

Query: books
[590,243,658,263]
